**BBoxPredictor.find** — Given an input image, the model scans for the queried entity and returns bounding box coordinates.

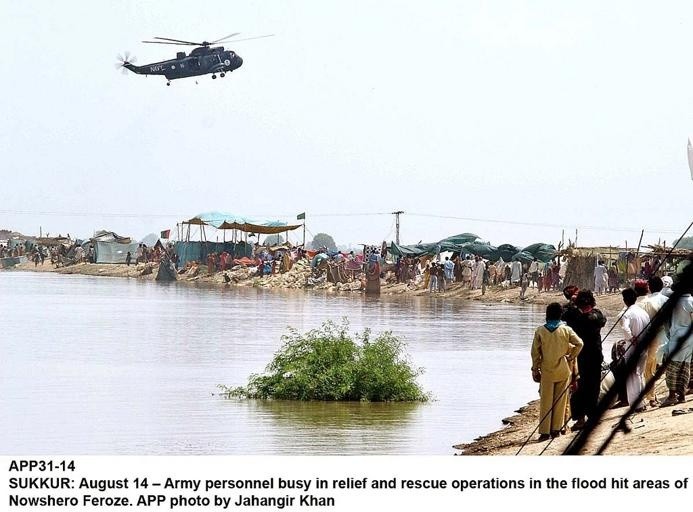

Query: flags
[297,213,305,220]
[160,230,171,239]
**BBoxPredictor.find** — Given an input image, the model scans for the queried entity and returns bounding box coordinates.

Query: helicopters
[113,32,276,86]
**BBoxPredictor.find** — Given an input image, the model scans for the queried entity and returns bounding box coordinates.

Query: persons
[0,243,651,297]
[529,264,692,443]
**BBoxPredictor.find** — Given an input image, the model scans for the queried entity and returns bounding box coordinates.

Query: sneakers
[571,423,584,430]
[674,396,685,402]
[659,398,679,408]
[538,430,560,441]
